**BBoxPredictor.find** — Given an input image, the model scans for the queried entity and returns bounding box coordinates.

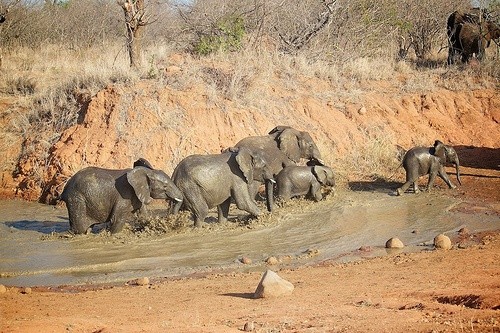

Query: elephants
[58,125,336,235]
[388,140,462,196]
[446,10,500,67]
